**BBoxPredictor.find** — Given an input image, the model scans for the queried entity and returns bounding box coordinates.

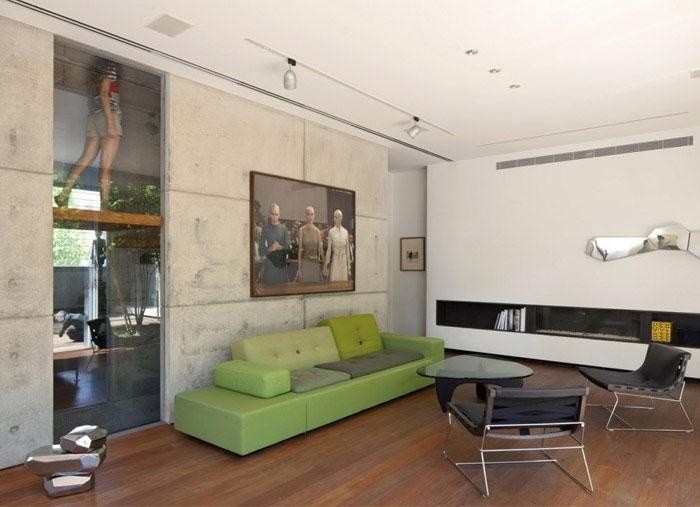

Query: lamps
[246,39,456,140]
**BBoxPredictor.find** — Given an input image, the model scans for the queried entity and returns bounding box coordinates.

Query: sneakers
[100,204,111,210]
[54,193,69,208]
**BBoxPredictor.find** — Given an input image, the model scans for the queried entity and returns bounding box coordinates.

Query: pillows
[314,349,423,378]
[214,362,292,398]
[289,367,351,393]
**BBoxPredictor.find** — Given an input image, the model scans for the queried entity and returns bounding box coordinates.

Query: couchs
[174,312,444,457]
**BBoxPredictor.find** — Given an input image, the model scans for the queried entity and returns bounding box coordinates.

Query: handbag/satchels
[267,251,286,268]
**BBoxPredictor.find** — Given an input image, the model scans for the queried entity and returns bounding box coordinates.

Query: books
[493,307,526,332]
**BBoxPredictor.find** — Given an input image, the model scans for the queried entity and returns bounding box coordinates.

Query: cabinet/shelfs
[436,299,700,383]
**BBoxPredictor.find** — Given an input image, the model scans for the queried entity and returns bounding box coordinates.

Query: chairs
[442,385,594,499]
[86,317,130,371]
[578,343,695,433]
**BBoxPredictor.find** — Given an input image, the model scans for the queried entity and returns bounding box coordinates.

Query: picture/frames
[250,169,355,298]
[399,237,426,271]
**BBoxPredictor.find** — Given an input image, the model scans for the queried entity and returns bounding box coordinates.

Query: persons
[325,209,351,282]
[54,58,122,209]
[298,206,324,283]
[258,203,290,285]
[90,230,106,281]
